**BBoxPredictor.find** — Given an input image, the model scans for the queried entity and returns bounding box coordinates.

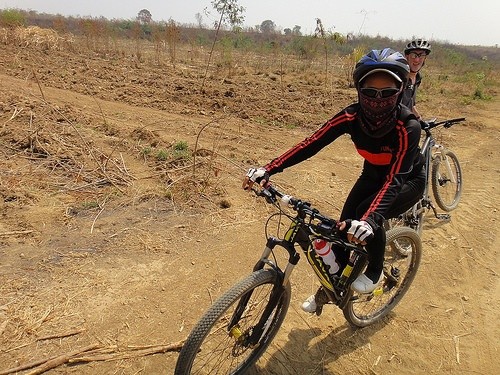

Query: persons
[242,48,427,312]
[398,38,432,123]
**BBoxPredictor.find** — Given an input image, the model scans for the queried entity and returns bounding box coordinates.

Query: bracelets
[417,115,421,119]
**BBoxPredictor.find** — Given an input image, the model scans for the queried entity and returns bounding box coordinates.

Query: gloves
[421,120,430,130]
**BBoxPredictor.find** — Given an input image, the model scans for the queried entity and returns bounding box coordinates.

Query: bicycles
[381,117,468,256]
[173,180,423,375]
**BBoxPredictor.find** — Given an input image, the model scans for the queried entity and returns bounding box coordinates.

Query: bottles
[314,239,340,275]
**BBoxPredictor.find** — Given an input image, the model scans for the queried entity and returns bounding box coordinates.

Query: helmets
[404,38,431,56]
[353,47,411,91]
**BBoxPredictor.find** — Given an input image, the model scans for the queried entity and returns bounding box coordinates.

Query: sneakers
[351,269,384,294]
[301,294,318,312]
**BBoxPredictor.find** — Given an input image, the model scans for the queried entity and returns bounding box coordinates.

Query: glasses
[409,52,427,58]
[360,87,400,98]
